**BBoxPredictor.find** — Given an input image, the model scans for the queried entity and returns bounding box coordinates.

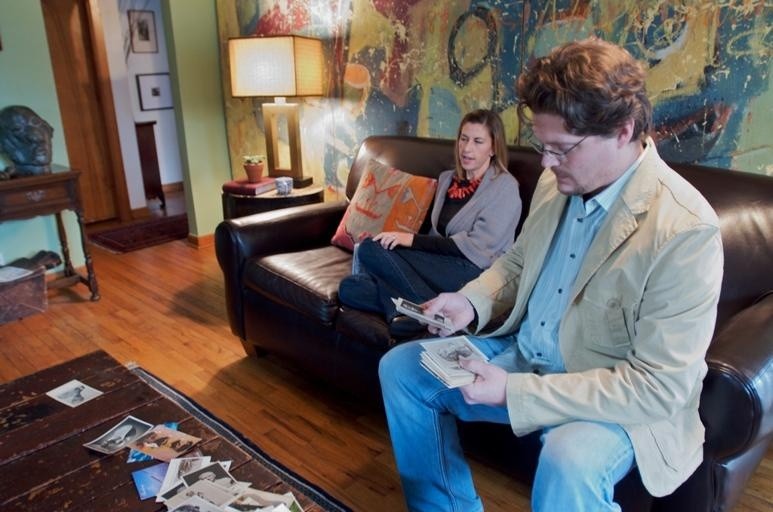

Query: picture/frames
[125,8,159,55]
[133,71,173,113]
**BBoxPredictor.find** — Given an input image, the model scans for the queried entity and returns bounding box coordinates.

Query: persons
[197,470,235,490]
[378,37,725,512]
[170,485,216,512]
[62,385,87,404]
[437,339,474,360]
[338,109,523,338]
[401,302,445,323]
[137,430,193,454]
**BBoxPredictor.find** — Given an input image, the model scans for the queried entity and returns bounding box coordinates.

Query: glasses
[526,131,593,162]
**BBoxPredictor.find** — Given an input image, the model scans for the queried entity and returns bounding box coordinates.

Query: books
[224,175,278,194]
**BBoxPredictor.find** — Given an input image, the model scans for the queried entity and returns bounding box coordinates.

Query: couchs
[212,130,773,511]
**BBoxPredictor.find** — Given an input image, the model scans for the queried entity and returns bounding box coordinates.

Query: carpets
[125,361,356,512]
[86,212,189,255]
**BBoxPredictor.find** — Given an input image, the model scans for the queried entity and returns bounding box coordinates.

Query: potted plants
[241,152,268,186]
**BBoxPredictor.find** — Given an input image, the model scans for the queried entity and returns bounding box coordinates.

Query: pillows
[328,157,439,260]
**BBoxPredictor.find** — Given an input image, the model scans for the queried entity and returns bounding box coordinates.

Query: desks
[0,349,331,512]
[220,182,327,224]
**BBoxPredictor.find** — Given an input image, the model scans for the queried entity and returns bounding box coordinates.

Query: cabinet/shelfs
[0,166,100,308]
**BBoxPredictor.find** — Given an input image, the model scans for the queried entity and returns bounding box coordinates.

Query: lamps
[225,34,329,185]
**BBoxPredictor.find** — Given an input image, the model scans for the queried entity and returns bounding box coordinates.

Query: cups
[275,176,293,196]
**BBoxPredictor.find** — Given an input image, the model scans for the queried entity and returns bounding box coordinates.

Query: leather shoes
[388,316,429,340]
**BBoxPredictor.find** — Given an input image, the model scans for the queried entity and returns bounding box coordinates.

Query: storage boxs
[0,254,50,323]
